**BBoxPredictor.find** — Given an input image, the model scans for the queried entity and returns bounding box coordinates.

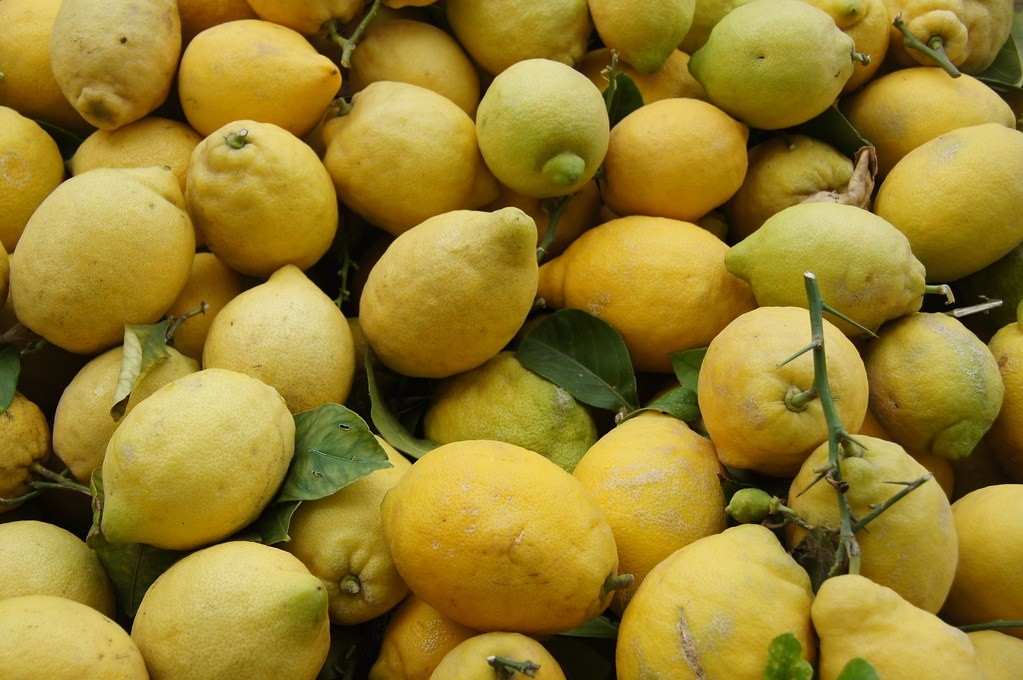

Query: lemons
[0,0,1023,680]
